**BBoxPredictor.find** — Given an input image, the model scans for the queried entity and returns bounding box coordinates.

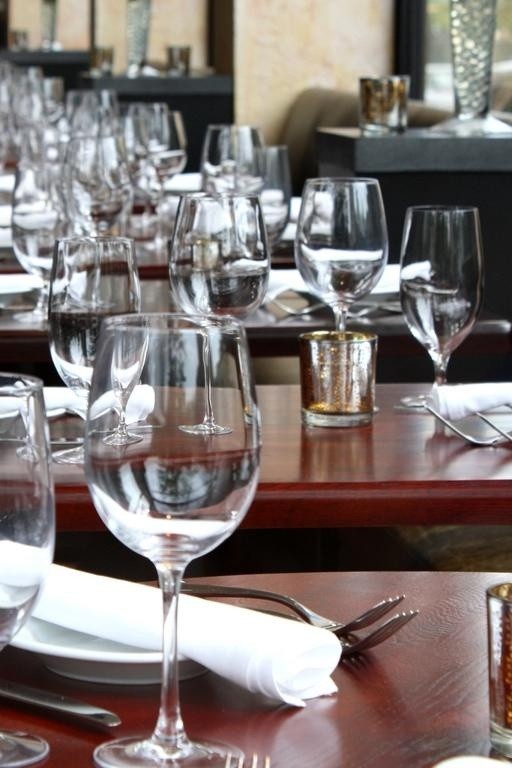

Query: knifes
[2,680,123,729]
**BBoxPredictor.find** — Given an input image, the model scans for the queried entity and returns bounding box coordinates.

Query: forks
[181,583,407,636]
[249,606,420,653]
[475,412,512,442]
[425,406,512,448]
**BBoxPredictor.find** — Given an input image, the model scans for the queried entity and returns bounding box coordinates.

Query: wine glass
[295,178,389,331]
[1,371,56,768]
[1,58,186,323]
[169,192,270,436]
[399,205,481,406]
[48,236,143,466]
[85,313,263,768]
[200,125,291,251]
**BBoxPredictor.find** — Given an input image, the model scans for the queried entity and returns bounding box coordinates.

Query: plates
[1,273,43,293]
[1,612,206,684]
[299,331,378,428]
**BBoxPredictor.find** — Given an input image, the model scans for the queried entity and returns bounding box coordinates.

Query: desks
[0,115,313,234]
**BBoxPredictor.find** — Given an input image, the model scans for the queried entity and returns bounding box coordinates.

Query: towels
[430,383,512,419]
[2,385,158,421]
[0,176,15,190]
[0,272,86,301]
[3,540,341,705]
[263,260,431,305]
[166,174,203,190]
[291,193,334,220]
[0,204,54,227]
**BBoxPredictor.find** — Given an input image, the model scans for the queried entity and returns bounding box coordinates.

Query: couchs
[276,87,456,196]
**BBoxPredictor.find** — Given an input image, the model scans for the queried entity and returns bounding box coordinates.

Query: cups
[485,581,512,754]
[88,46,115,79]
[168,45,191,78]
[7,29,31,55]
[357,76,410,136]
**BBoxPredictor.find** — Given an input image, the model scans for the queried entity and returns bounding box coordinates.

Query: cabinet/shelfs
[316,119,512,382]
[12,38,233,168]
